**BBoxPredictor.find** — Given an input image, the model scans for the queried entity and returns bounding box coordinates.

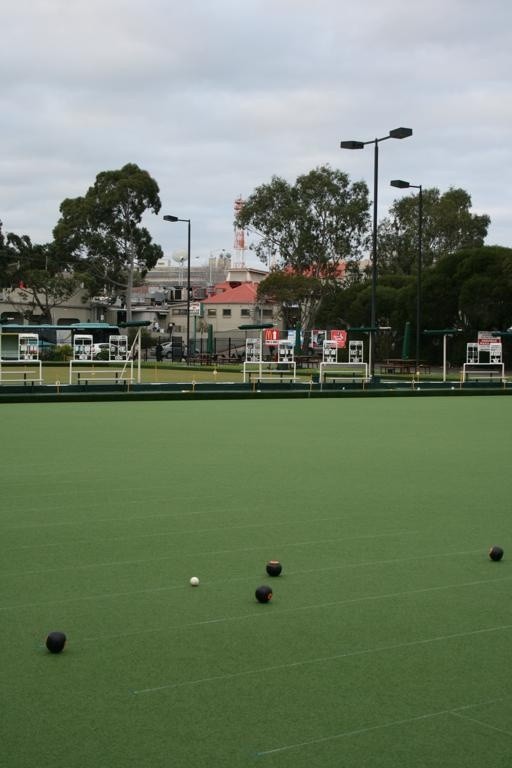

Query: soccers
[266,560,281,576]
[255,586,272,602]
[489,546,503,561]
[46,632,66,653]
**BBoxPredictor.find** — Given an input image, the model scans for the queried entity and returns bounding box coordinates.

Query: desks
[381,358,415,365]
[293,353,314,367]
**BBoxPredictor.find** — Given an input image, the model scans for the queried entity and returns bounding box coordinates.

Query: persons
[341,348,349,362]
[154,341,164,362]
[271,349,278,362]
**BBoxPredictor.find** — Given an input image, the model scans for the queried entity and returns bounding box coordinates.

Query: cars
[84,343,124,359]
[151,341,200,359]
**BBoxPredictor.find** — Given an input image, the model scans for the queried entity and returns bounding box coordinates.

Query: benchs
[240,370,373,389]
[0,369,137,393]
[459,370,509,389]
[301,359,320,368]
[380,363,429,374]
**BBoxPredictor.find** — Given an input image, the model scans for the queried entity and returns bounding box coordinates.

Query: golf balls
[190,577,198,585]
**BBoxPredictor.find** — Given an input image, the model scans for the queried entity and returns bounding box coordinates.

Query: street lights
[390,178,423,367]
[340,127,412,371]
[164,214,191,357]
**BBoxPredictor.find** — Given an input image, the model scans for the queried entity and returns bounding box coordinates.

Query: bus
[0,323,120,360]
